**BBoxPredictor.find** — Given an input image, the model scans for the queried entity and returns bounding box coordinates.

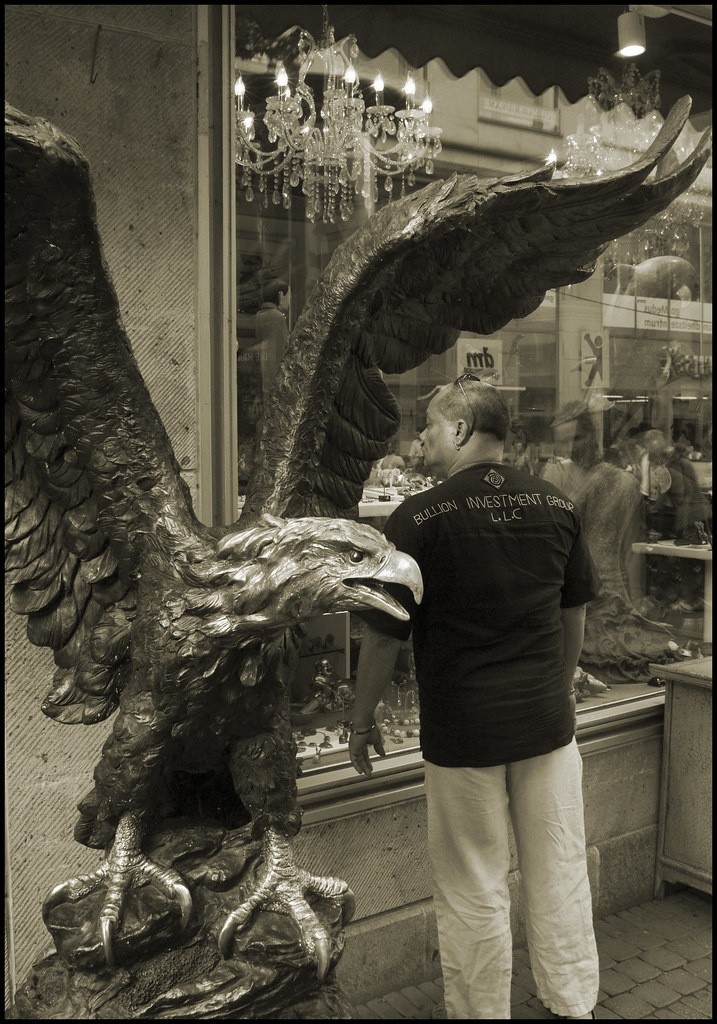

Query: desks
[646,656,712,898]
[632,540,713,646]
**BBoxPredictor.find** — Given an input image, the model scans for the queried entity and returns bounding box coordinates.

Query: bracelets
[349,721,377,734]
[568,689,576,695]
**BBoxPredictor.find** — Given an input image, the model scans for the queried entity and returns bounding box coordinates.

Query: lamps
[616,10,646,56]
[223,2,440,223]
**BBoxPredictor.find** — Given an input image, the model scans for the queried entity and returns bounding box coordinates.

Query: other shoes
[560,1009,597,1020]
[432,1001,447,1019]
[672,596,704,614]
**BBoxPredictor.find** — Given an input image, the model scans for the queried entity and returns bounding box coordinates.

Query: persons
[379,435,409,487]
[515,441,535,477]
[254,279,292,405]
[348,373,601,1019]
[536,399,648,625]
[409,429,430,477]
[606,426,712,613]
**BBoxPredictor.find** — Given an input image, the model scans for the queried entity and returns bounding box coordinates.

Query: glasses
[454,373,481,436]
